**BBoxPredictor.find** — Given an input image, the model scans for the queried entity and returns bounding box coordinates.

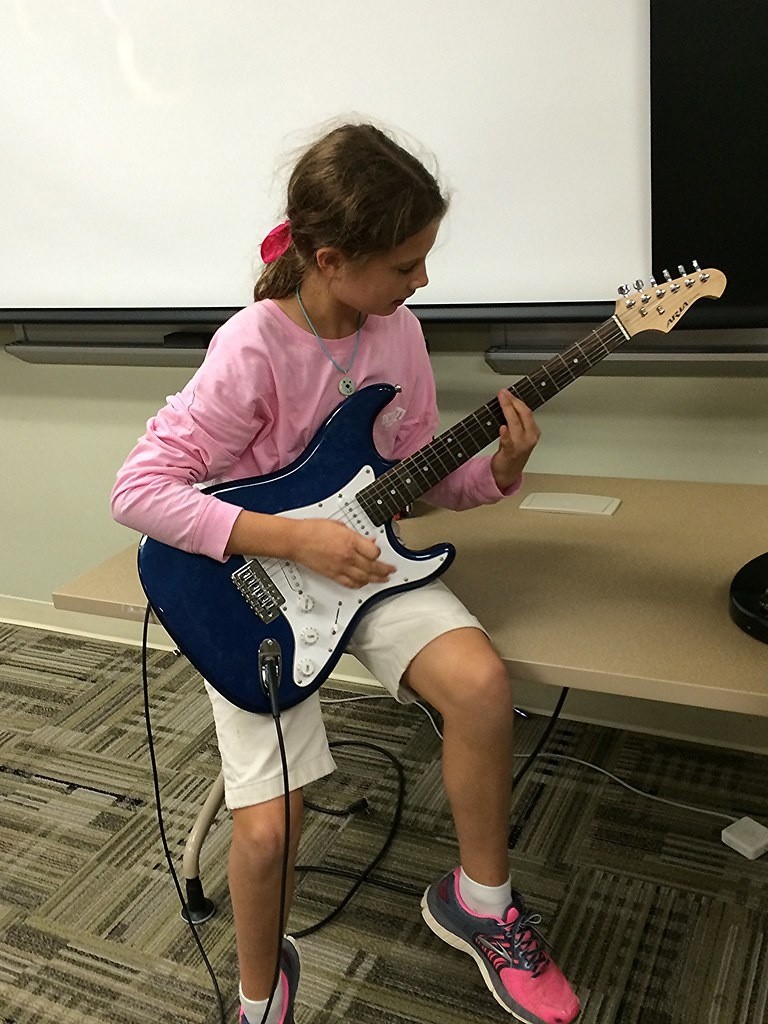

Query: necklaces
[292,276,364,401]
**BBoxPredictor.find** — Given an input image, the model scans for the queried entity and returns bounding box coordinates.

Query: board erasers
[162,329,213,349]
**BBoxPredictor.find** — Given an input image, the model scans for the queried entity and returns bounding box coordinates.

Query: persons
[108,120,586,1024]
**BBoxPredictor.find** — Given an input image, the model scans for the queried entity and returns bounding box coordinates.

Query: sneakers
[419,865,583,1024]
[237,934,300,1024]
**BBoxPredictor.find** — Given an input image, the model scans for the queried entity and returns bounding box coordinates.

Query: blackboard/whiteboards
[3,2,766,369]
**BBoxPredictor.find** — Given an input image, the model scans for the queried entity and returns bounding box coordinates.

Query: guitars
[137,257,728,716]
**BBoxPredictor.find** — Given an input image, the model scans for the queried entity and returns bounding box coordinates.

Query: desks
[46,439,768,926]
